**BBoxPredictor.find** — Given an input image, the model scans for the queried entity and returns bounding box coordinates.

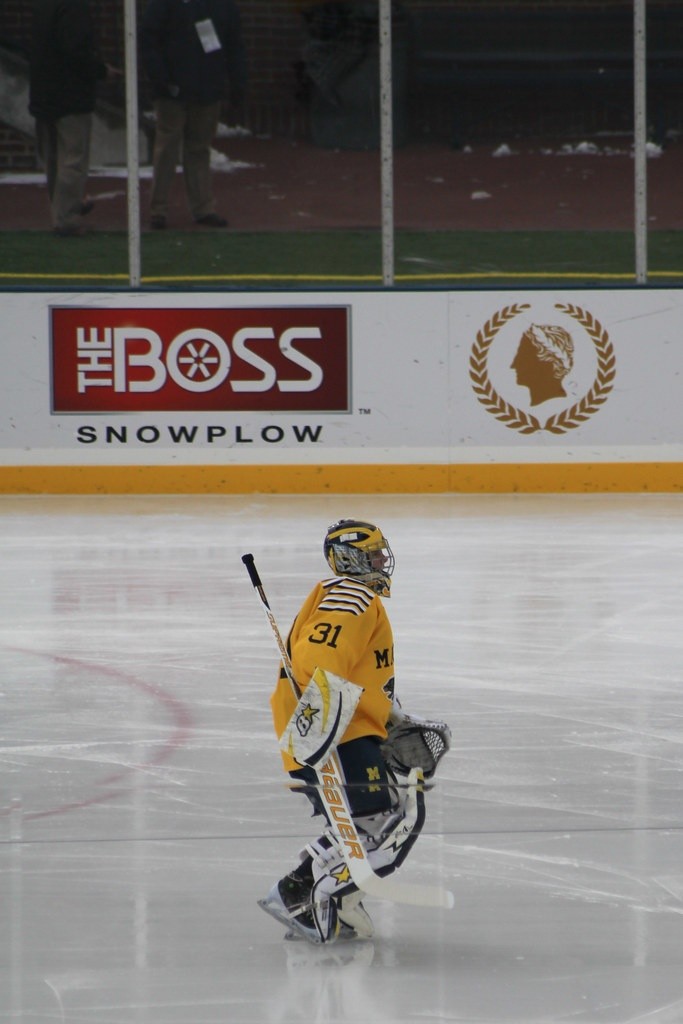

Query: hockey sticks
[241,553,457,913]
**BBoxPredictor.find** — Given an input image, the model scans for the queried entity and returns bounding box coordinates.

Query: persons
[258,520,427,946]
[26,0,106,233]
[145,0,241,230]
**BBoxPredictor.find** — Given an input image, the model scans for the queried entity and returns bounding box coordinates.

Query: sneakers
[256,871,335,944]
[339,889,374,938]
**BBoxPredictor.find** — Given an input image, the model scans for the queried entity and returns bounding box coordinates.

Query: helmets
[323,519,396,599]
